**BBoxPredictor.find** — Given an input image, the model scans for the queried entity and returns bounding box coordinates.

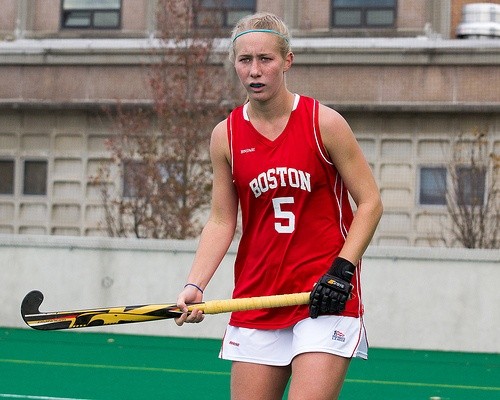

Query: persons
[175,12,383,400]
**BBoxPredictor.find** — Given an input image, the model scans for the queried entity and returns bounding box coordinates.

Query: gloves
[308,256,357,320]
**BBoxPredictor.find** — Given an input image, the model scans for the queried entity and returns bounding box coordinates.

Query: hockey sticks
[21,290,352,331]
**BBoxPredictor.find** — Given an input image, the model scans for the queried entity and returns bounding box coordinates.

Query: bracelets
[184,283,203,293]
